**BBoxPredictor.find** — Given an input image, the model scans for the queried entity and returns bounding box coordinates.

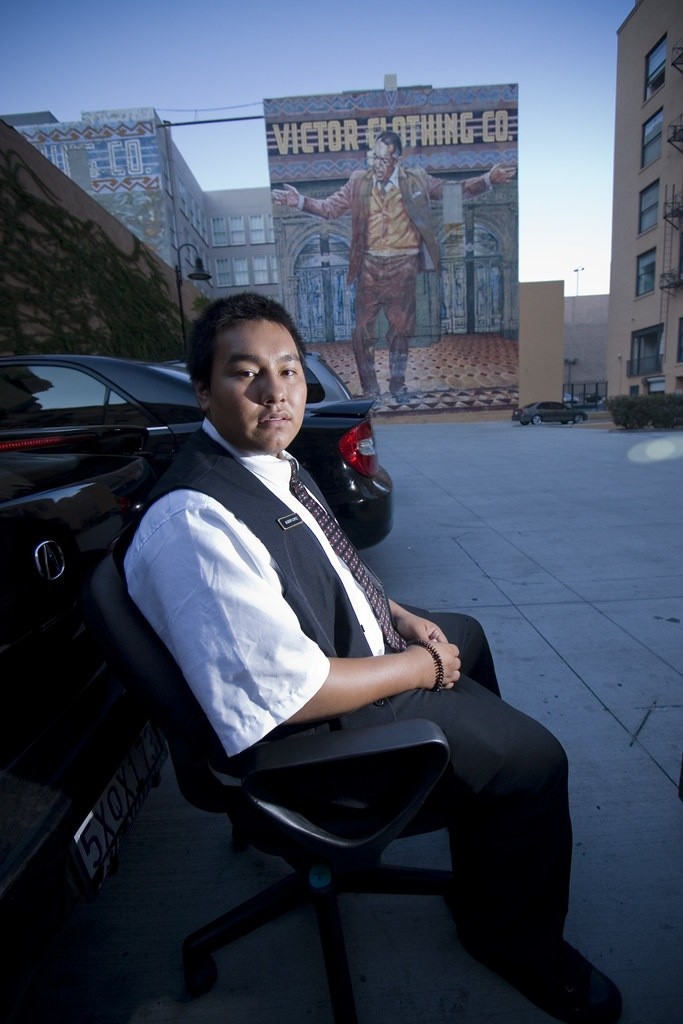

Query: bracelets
[407,639,443,692]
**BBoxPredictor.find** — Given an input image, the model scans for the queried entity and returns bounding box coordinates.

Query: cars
[584,392,606,405]
[512,401,589,425]
[564,392,579,403]
[0,350,391,1023]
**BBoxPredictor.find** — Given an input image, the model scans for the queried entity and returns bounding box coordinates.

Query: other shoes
[522,941,623,1024]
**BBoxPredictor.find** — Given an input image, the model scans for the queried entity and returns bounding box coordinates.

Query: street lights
[175,244,211,354]
[574,266,584,296]
[565,357,578,394]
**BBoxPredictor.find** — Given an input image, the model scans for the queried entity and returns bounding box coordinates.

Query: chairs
[96,517,491,1024]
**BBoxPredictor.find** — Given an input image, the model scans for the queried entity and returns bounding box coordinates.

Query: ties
[289,458,413,652]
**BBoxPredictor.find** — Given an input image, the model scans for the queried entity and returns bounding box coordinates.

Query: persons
[122,292,625,1024]
